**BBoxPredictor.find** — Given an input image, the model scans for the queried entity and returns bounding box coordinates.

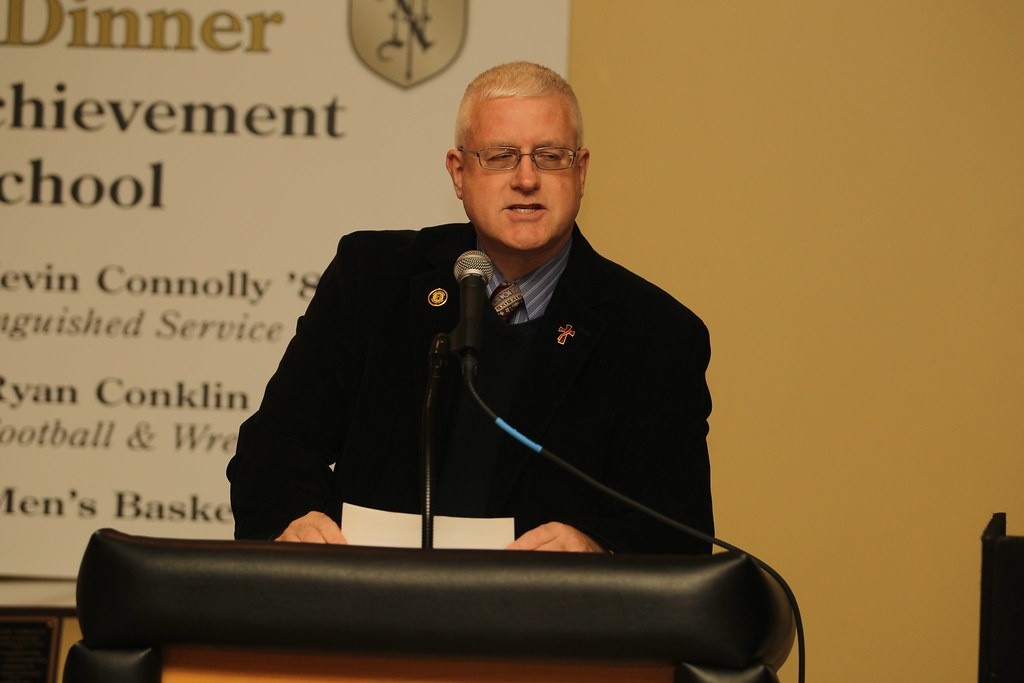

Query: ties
[490,281,524,323]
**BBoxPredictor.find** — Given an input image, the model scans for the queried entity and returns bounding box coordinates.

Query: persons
[226,63,716,556]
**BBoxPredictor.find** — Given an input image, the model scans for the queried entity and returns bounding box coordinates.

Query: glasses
[457,146,581,170]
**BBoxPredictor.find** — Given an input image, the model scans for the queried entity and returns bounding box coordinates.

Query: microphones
[454,251,494,379]
